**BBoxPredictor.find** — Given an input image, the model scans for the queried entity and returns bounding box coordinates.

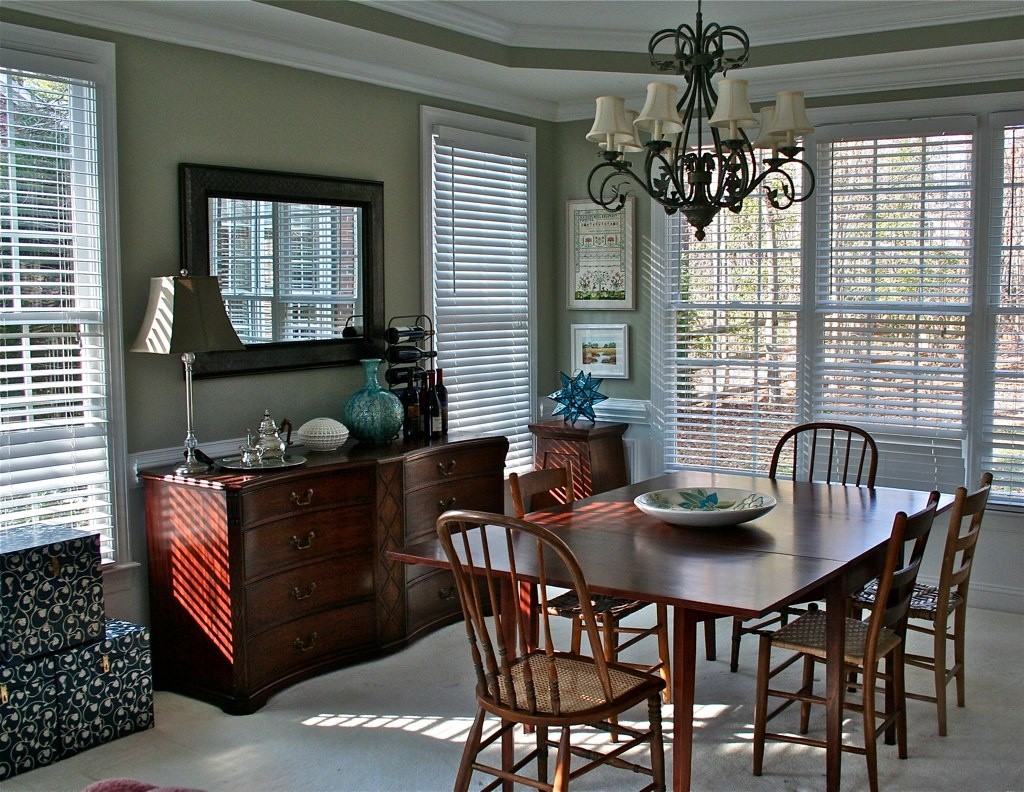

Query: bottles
[384,326,435,344]
[344,359,405,443]
[418,372,432,441]
[392,387,429,400]
[426,370,442,441]
[385,346,437,363]
[436,369,448,435]
[385,366,435,384]
[342,326,364,337]
[402,368,420,440]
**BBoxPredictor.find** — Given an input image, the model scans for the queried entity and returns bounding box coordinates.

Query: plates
[633,487,778,526]
[215,455,307,469]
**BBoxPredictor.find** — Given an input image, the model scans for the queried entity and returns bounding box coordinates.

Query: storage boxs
[0,523,154,781]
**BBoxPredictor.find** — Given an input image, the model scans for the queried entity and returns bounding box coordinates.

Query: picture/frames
[564,196,637,312]
[570,324,631,380]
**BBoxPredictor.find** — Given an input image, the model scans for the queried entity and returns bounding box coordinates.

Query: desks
[528,419,630,511]
[384,471,957,792]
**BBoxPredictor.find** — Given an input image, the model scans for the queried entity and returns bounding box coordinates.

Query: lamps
[586,0,815,242]
[128,270,245,476]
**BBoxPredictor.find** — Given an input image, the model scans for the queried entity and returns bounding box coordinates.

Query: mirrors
[175,162,385,381]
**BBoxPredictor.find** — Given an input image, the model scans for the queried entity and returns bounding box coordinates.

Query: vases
[343,359,405,444]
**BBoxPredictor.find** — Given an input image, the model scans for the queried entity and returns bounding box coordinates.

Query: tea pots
[245,408,294,458]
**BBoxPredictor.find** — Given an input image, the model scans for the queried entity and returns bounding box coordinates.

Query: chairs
[438,423,1009,792]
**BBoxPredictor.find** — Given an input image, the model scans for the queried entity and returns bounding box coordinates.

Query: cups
[239,445,264,466]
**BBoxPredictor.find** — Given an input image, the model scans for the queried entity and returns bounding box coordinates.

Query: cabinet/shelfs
[137,434,510,715]
